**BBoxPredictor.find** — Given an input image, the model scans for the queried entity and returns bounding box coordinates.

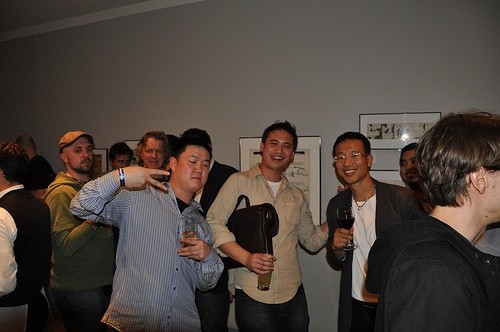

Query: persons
[110,128,241,332]
[400,142,434,215]
[0,136,57,332]
[42,131,115,332]
[206,121,349,332]
[375,107,500,332]
[326,133,428,332]
[68,136,224,332]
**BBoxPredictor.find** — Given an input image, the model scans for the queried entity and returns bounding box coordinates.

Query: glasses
[333,152,368,163]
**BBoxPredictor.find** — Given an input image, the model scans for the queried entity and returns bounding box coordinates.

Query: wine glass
[337,206,359,251]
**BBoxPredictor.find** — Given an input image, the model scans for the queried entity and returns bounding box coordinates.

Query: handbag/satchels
[220,194,279,269]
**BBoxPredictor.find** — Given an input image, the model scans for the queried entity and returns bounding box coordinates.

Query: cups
[257,252,274,291]
[178,213,199,248]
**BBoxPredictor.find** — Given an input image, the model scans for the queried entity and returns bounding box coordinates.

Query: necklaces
[353,184,376,211]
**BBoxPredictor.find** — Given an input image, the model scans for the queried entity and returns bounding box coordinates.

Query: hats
[58,131,96,151]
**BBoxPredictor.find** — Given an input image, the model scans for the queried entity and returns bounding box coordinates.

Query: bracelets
[119,168,125,186]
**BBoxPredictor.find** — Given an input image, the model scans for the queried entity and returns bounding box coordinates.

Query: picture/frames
[358,112,441,150]
[124,139,140,166]
[89,149,109,178]
[239,135,322,235]
